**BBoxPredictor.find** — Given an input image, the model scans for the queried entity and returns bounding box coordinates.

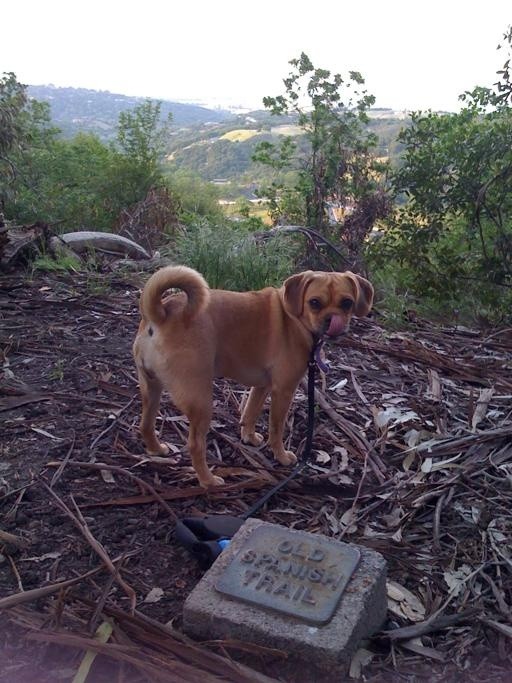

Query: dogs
[132,263,374,489]
